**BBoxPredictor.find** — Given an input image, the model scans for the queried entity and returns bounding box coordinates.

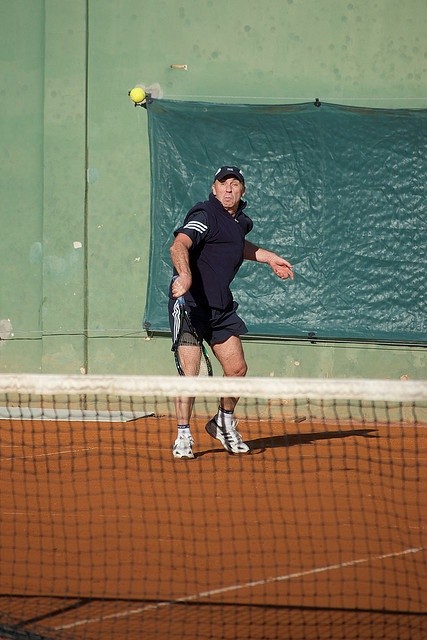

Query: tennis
[130,87,145,103]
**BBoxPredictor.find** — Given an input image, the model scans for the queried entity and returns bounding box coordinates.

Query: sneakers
[172,436,195,459]
[205,415,251,456]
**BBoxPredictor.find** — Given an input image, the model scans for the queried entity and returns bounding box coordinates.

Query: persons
[168,166,294,459]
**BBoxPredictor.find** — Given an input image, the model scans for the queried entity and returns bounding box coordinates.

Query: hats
[214,166,245,184]
